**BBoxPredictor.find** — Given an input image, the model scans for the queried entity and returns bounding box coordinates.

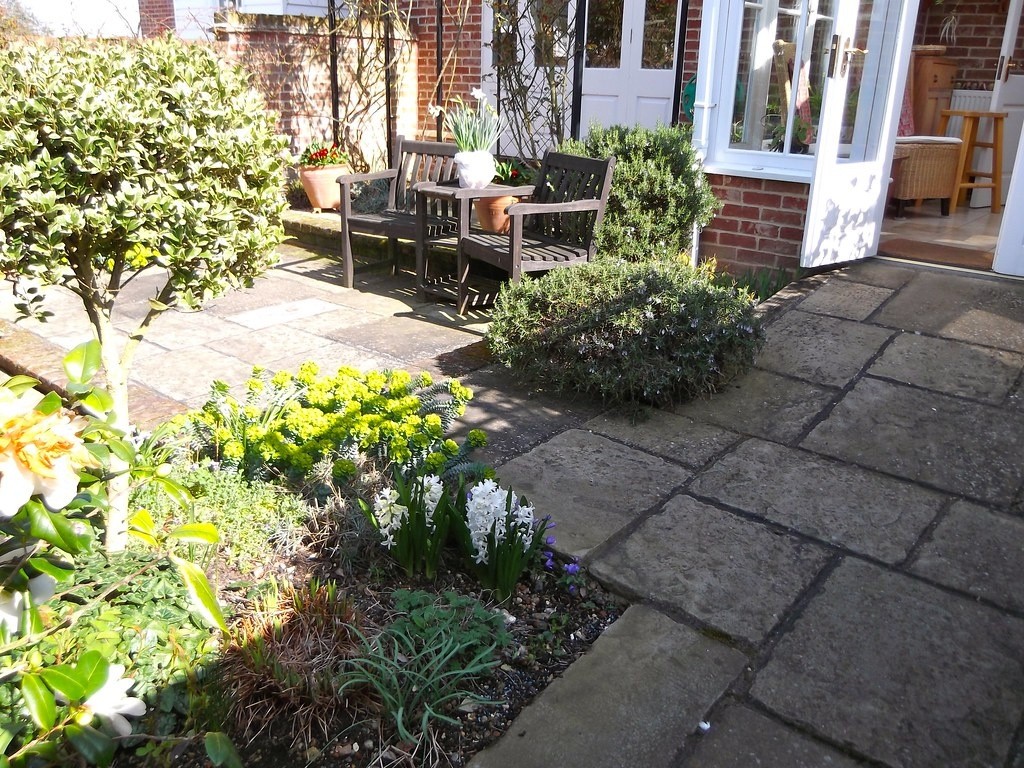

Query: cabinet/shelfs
[914,55,959,136]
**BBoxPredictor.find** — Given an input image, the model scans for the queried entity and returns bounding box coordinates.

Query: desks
[916,110,1010,214]
[418,183,512,306]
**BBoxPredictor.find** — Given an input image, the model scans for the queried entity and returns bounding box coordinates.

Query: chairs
[891,52,962,218]
[449,146,616,316]
[772,38,911,219]
[335,134,461,304]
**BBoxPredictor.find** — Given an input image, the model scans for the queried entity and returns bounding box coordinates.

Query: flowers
[429,86,511,152]
[297,140,349,168]
[493,157,535,187]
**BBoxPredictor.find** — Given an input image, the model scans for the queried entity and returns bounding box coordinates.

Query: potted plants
[766,117,810,154]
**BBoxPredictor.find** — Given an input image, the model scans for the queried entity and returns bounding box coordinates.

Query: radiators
[945,89,993,169]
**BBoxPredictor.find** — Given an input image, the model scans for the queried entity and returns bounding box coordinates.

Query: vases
[454,150,496,189]
[472,197,524,234]
[297,163,350,214]
